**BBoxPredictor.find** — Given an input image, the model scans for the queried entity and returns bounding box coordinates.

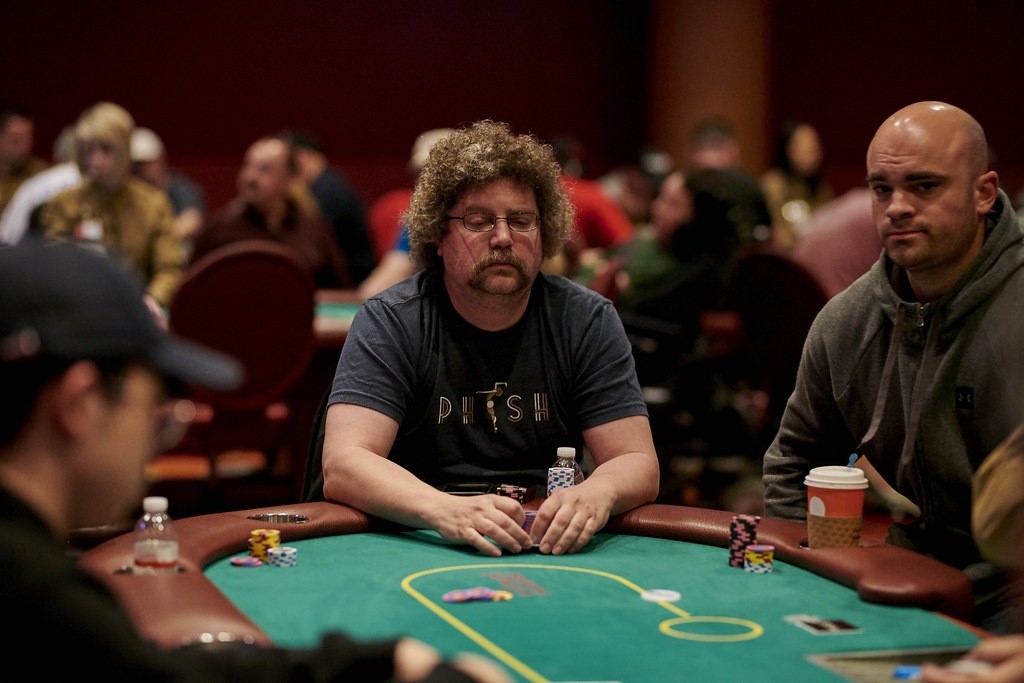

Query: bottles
[133,497,179,575]
[551,447,585,488]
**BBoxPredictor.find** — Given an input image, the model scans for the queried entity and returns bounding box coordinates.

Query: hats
[0,240,242,394]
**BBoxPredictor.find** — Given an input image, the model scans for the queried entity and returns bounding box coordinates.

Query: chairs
[157,234,319,516]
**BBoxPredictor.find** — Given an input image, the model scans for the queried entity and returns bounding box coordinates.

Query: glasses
[445,211,547,235]
[33,379,190,451]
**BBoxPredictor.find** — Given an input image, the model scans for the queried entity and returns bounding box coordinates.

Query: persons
[536,112,884,445]
[0,104,459,331]
[762,102,1023,683]
[320,118,659,554]
[0,240,510,683]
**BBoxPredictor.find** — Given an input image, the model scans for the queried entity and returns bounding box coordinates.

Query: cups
[803,465,870,548]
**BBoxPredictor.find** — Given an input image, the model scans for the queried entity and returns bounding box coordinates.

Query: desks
[121,283,369,345]
[81,493,1001,683]
